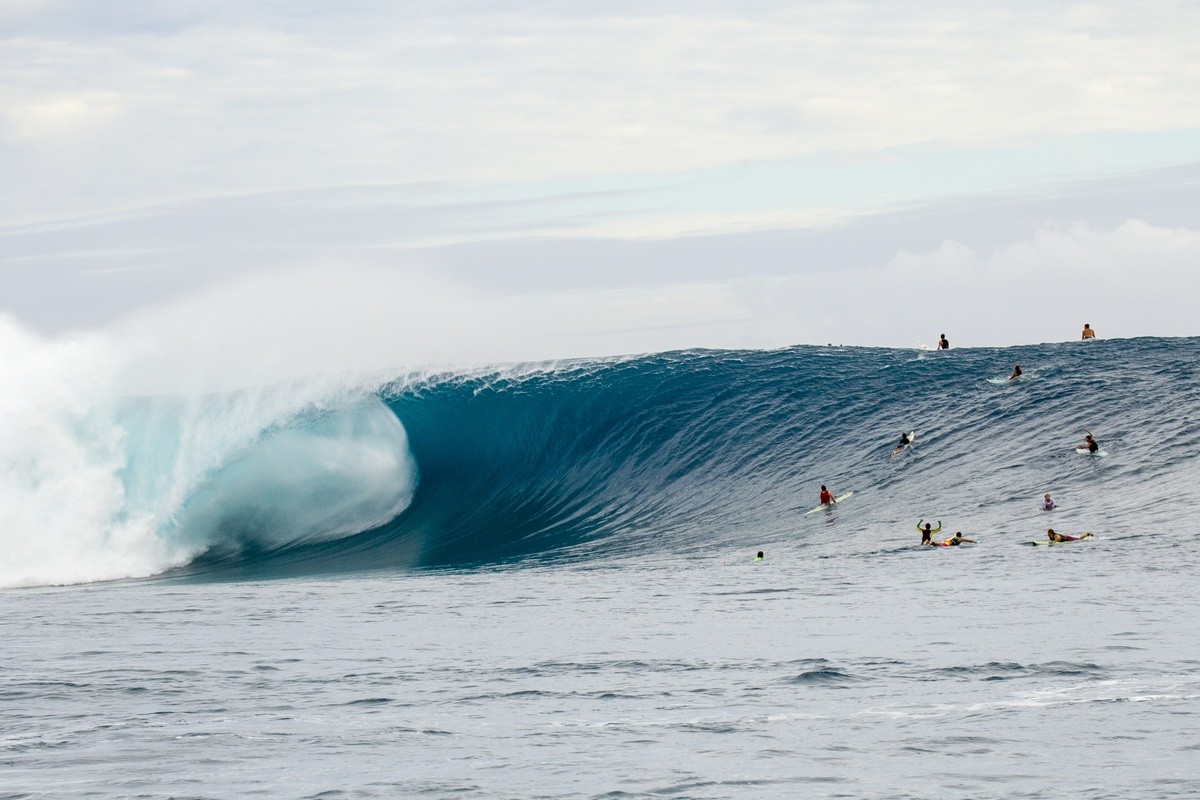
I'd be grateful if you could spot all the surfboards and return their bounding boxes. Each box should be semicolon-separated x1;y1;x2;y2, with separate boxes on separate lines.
1076;448;1109;457
803;491;853;514
891;431;915;457
986;376;1026;384
920;344;937;351
1035;540;1069;544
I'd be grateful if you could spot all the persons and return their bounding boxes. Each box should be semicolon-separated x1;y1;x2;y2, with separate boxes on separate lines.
1009;366;1022;380
922;532;976;546
1079;430;1098;454
937;334;949;350
1048;529;1094;542
916;520;941;545
1082;324;1095;340
820;485;836;505
892;433;910;457
1042;494;1056;511
754;551;763;561
